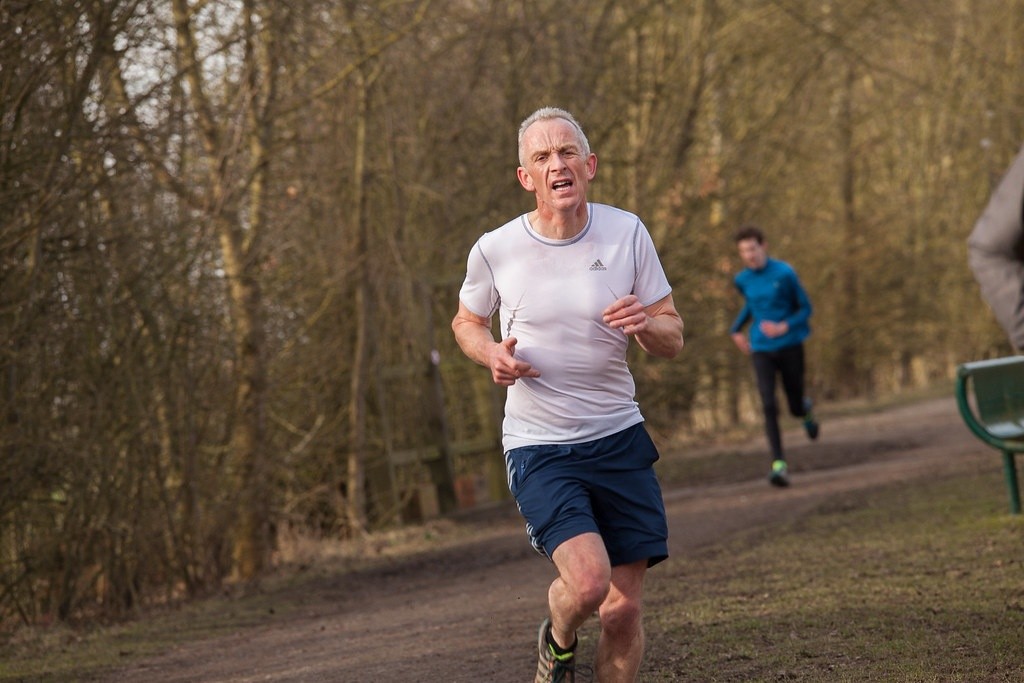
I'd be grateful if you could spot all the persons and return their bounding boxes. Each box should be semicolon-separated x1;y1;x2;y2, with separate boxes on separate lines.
966;147;1024;356
730;228;819;486
453;108;683;683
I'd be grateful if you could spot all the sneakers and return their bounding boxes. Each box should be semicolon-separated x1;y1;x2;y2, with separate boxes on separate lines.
534;616;581;683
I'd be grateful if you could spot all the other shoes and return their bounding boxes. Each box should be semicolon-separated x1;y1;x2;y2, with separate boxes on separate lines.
767;460;792;487
802;400;819;440
